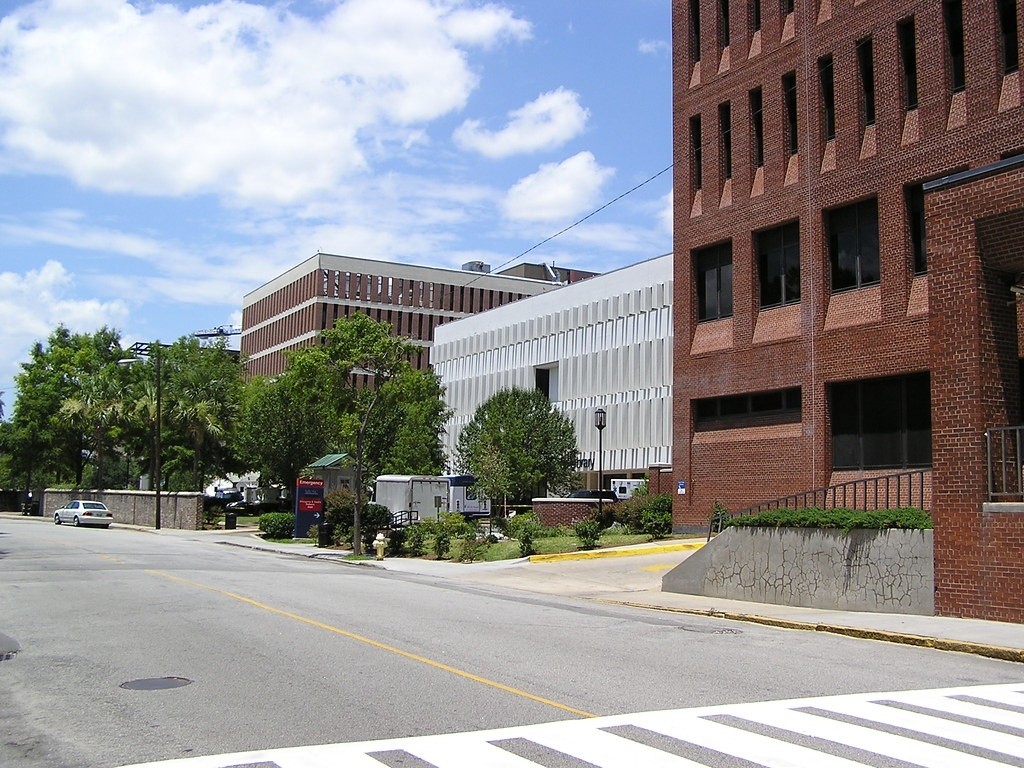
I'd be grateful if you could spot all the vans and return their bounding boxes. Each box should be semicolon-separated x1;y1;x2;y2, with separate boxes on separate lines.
216;488;245;504
567;490;619;503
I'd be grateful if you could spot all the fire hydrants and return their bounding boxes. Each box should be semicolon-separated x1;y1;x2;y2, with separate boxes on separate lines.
374;533;387;560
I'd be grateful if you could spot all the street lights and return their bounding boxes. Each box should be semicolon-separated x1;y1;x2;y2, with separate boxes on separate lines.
594;408;607;516
118;359;163;529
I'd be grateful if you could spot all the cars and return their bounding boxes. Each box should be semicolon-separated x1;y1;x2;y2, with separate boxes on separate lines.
226;500;283;515
53;501;113;530
